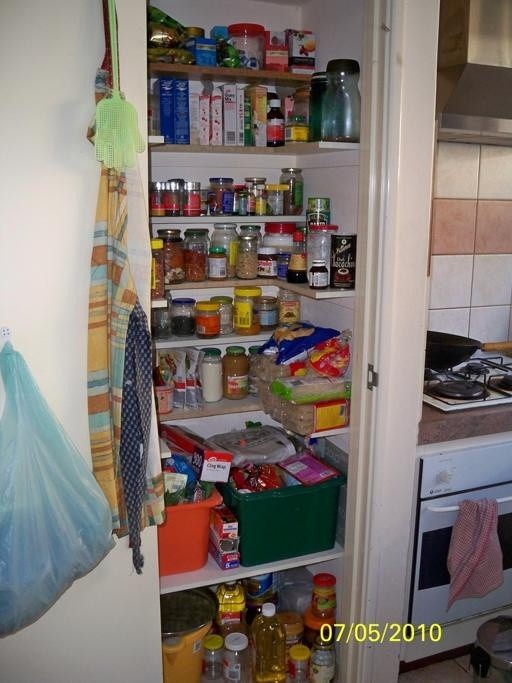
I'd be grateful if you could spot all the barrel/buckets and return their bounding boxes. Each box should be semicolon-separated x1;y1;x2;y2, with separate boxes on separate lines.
215;580;249;637
159;588;218;683
249;602;288;683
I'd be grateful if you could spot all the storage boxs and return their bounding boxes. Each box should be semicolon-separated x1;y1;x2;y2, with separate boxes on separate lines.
213;432;346;567
157;487;224;577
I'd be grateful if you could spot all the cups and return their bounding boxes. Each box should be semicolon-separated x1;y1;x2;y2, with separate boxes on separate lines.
336;267;350;281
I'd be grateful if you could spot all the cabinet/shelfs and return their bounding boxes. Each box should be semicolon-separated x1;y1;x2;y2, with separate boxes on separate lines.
119;1;395;683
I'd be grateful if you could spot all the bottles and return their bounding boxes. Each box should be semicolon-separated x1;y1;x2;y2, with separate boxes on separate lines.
226;23;267;70
292;87;310;124
210;223;338;290
310;70;347;139
322;57;362;141
237;191;247;215
266;99;285;147
169;296;197;337
254;183;267;215
209;295;233;334
248;344;266;396
202;570;337;682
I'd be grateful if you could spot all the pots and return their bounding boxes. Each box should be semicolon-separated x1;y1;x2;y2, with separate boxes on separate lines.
425;328;482;371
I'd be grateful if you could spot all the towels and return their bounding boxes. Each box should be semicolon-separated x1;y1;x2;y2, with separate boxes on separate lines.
446;498;504;611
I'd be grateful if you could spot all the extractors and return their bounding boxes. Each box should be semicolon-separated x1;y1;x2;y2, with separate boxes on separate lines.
434;0;512;145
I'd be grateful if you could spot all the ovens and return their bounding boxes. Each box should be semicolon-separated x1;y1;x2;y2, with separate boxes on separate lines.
405;430;512;668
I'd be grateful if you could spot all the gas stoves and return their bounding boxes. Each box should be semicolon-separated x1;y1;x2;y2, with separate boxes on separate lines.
425;354;511;413
